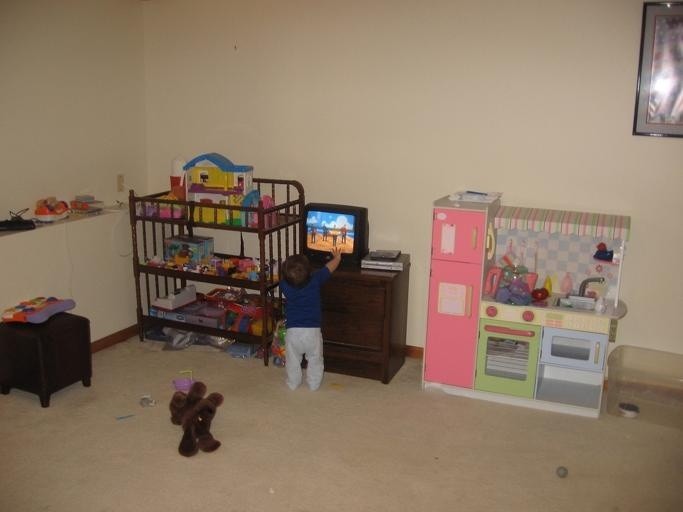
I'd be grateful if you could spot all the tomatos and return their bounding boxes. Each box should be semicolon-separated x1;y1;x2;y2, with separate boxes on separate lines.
532;288;549;300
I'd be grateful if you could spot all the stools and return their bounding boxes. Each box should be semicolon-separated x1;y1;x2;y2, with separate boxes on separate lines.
0;311;92;407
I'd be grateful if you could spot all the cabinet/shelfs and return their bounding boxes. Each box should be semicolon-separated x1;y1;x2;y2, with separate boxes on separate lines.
301;253;411;384
128;174;305;367
421;192;500;390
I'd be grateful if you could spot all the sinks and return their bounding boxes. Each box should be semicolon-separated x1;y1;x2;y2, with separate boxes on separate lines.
553;293;611;316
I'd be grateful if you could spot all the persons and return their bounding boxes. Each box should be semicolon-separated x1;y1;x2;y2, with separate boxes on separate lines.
331;224;337;246
340;224;346;244
279;246;341;392
321;222;327;241
308;223;316;243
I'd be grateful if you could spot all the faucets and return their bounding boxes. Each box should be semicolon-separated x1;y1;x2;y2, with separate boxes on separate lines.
578;276;604;296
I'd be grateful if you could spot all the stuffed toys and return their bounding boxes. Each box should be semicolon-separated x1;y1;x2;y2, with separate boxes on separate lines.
168;380;223;456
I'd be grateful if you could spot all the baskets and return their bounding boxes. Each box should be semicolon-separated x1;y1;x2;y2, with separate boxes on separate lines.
207;289;235;306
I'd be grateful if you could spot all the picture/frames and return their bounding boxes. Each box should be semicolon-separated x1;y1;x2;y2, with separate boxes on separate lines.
632;1;683;138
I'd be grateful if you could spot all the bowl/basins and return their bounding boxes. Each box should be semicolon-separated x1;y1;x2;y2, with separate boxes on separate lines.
173;377;196;391
503;264;528;287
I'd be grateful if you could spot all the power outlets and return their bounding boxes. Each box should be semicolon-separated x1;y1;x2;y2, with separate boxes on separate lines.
117;175;125;192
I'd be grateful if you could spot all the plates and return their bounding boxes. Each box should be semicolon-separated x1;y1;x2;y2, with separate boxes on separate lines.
34;210;71;222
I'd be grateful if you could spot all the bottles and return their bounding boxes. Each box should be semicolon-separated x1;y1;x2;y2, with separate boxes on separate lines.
595;296;606;315
544;275;552;297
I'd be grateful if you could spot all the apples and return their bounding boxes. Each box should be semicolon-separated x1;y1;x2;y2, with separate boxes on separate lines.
37;197;57;210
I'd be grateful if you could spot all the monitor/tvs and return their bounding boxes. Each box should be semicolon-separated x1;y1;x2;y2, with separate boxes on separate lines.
302;203;369;268
